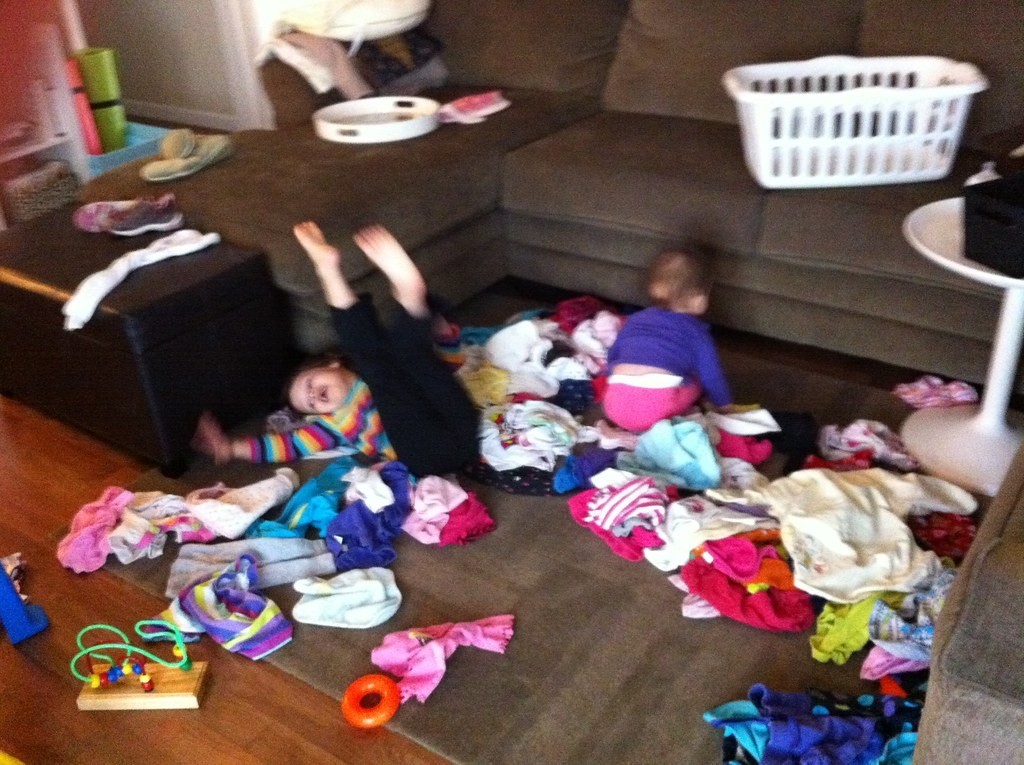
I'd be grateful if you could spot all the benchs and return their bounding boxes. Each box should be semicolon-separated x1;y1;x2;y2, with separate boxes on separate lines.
0;197;288;478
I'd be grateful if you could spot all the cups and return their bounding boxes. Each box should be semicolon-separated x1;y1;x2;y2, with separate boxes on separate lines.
65;47;129;156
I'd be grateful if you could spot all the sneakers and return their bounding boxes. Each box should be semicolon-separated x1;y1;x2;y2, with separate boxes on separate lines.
73;200;138;233
109;192;185;237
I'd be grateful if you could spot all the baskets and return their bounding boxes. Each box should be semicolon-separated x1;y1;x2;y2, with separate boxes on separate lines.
722;53;986;192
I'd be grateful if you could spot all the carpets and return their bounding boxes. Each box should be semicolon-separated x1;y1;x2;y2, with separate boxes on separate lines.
52;293;990;765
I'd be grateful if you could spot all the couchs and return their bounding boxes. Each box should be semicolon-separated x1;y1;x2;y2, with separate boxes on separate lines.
72;0;1024;391
912;444;1024;765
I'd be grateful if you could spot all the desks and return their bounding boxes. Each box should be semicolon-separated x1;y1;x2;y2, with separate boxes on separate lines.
901;195;1024;498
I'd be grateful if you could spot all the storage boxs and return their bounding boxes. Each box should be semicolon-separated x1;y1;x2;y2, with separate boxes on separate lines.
85;122;173;179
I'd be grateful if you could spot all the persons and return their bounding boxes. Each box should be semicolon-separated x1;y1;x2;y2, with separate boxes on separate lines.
603;248;730;431
191;223;480;478
